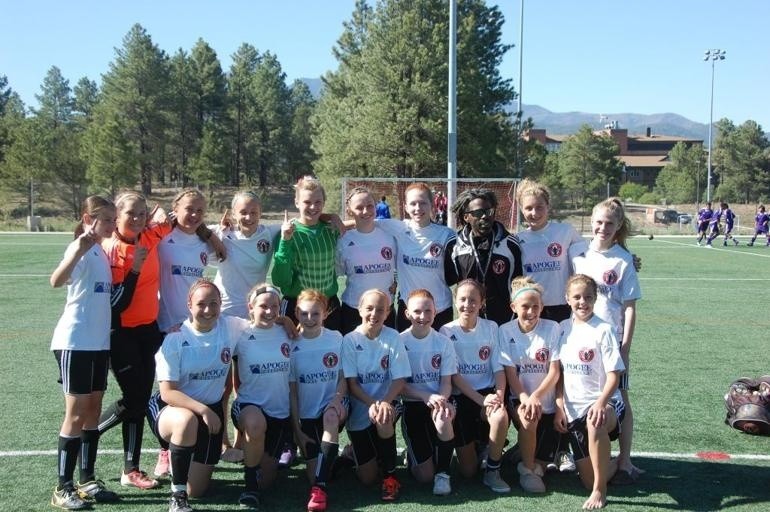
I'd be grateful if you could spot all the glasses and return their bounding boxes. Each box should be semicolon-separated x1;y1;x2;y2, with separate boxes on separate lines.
464;206;495;218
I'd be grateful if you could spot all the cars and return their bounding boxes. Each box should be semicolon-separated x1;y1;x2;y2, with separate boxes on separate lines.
678;213;692;224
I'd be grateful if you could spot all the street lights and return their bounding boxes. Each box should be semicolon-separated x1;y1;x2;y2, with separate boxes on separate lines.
703;48;726;203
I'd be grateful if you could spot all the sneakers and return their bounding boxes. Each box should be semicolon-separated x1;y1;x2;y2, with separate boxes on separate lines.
610;467;639;484
51;481;89;510
381;476;401;500
238;489;260;510
220;446;244;463
697;237;770;249
279;443;297;465
401;444;576;495
345;443;354;460
154;448;169;476
169;490;193;512
76;477;118;503
307;485;328;512
121;468;159;489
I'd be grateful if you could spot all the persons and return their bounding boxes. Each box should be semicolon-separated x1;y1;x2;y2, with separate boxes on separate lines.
146;274;629;511
146;175;645;476
696;203;770;248
48;189;228;510
